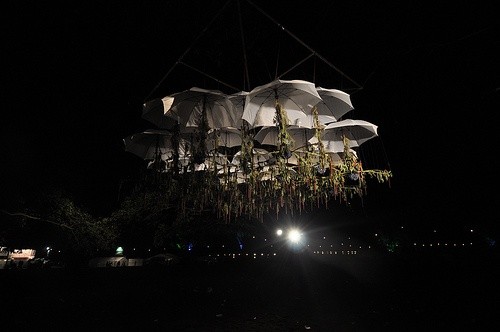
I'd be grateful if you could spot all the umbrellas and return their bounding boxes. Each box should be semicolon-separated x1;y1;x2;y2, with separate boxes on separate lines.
122;78;381;192
